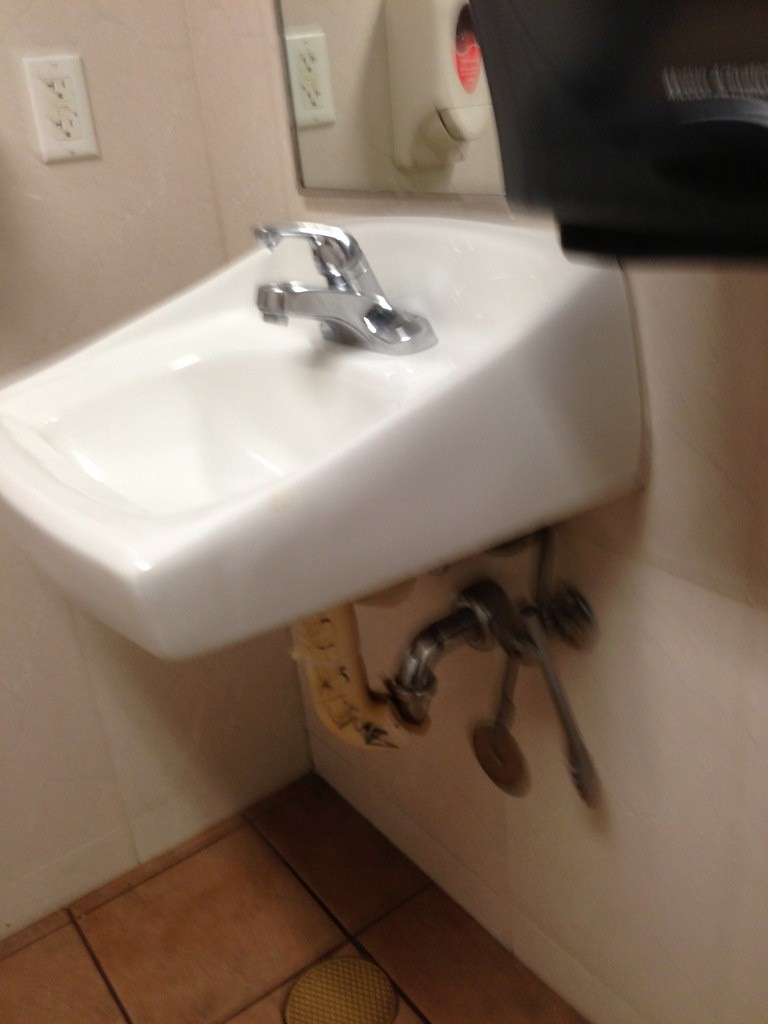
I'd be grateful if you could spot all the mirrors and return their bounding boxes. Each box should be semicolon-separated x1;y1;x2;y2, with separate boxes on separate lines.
276;0;506;199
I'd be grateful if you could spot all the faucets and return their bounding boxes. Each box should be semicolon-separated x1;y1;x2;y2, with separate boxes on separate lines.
252;222;438;356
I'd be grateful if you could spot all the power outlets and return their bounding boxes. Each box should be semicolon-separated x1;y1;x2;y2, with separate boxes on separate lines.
285;31;335;127
24;53;98;163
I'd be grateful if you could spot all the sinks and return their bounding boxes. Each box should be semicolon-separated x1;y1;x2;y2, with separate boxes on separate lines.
2;211;644;660
